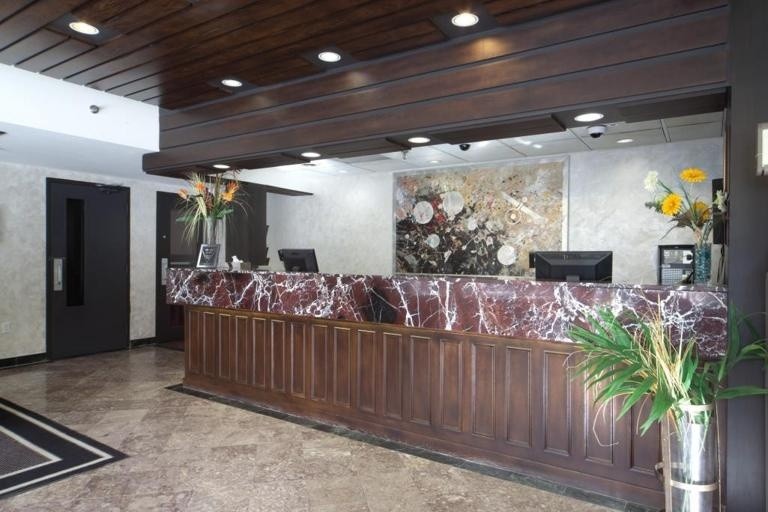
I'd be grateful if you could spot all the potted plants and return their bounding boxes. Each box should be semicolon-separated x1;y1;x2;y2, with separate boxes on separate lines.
557;298;767;512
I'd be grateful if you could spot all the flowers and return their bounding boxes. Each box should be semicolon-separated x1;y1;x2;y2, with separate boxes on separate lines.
172;165;253;249
644;166;731;249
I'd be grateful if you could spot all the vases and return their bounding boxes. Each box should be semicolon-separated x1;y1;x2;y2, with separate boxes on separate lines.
692;244;711;285
204;216;218;245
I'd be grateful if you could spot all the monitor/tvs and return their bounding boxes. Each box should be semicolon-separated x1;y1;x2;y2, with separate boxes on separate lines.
278;249;319;272
528;250;613;283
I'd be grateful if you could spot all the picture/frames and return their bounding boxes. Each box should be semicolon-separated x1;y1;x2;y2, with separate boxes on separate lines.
195;243;221;269
389;152;572;281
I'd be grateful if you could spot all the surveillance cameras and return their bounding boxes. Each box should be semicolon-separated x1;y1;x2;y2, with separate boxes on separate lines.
459;144;471;151
587;127;606;140
